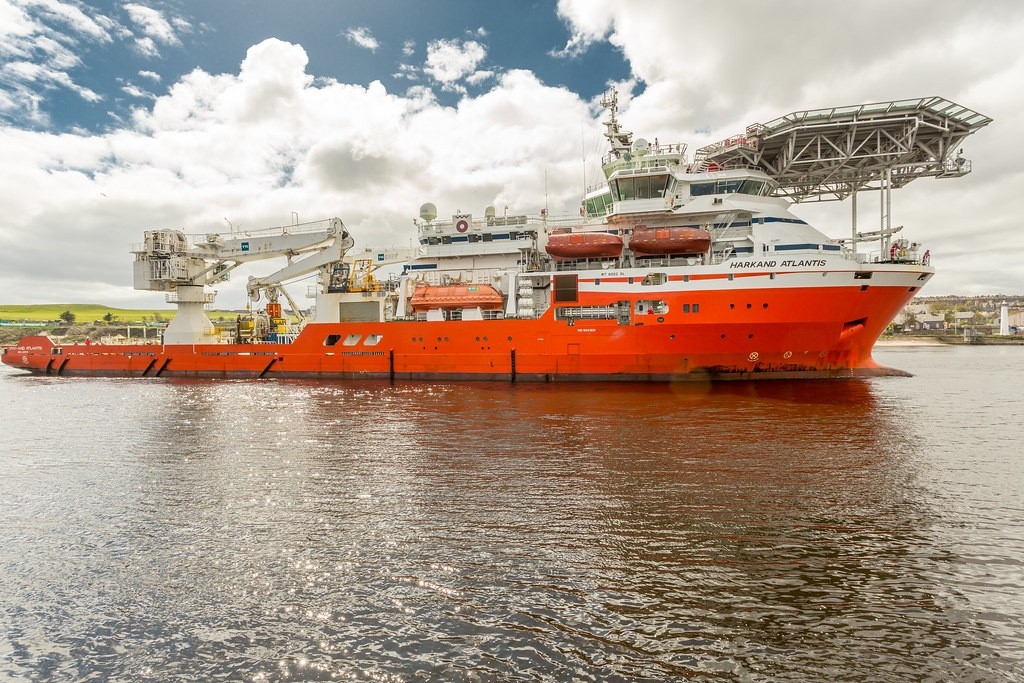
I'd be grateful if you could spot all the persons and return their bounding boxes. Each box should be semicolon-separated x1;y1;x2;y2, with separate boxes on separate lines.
922;250;930;265
356;267;365;287
890;240;902;264
85;336;91;345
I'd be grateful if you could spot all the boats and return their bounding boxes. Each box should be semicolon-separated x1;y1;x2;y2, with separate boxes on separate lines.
2;83;994;382
